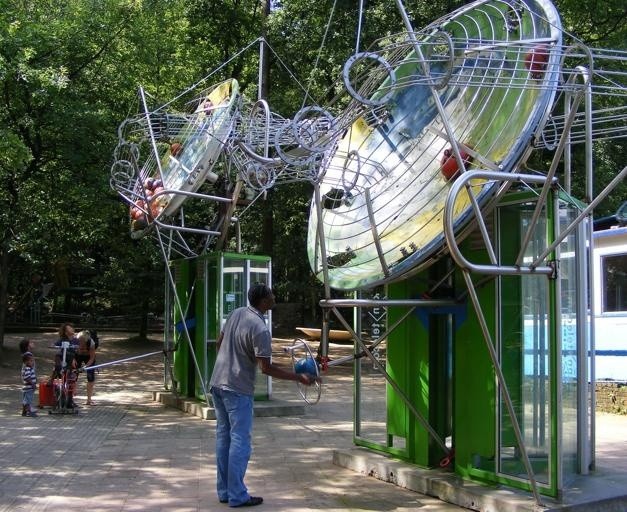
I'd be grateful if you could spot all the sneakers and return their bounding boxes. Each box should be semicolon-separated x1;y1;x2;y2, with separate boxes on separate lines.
22;410;38;417
219;495;264;508
67;401;98;408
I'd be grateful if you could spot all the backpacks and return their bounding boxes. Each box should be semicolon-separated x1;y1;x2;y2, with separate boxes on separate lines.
86;328;99;351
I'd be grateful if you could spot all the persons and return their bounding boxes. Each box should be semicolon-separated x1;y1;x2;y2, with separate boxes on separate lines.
207;282;316;508
19;323;96;418
